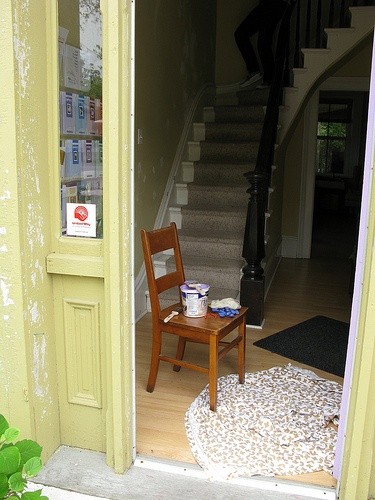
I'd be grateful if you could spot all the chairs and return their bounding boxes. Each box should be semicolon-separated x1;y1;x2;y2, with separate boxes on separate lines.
139;221;248;412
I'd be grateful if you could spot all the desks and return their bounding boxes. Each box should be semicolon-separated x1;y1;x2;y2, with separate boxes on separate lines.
314;178;348;222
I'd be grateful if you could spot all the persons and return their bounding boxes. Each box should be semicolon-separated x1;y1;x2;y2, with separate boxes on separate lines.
234;0;282;90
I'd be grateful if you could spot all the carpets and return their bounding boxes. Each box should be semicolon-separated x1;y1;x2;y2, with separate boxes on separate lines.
253;315;350;380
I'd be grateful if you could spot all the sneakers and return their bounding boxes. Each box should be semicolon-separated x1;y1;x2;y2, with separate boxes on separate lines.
255;78;270;89
239;71;262;88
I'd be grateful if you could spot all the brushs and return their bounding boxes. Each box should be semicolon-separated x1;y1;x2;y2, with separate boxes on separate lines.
163;306;184;323
184;279;206;296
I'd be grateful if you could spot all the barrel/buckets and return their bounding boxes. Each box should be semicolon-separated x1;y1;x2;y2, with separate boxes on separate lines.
180;284;210;318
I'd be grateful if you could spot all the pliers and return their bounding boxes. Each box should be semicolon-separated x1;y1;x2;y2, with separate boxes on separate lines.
204;309;218;320
